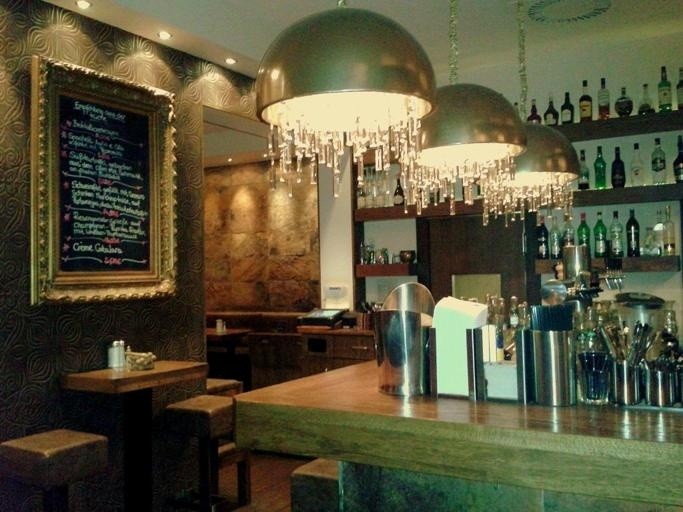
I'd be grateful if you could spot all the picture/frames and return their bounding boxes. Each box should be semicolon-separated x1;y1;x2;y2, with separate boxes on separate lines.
28;48;180;319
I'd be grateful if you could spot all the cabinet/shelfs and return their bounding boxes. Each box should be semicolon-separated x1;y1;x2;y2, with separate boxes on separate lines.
349;143;428;276
525;109;682;302
297;322;376;370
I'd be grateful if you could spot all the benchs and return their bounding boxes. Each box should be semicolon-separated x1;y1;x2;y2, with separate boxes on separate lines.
205;309;303;356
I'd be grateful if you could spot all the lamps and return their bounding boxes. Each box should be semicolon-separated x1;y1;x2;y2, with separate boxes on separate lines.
254;1;437;198
405;0;527;220
488;0;580;233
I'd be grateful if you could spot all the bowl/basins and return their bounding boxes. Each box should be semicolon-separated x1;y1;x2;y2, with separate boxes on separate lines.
398;249;417;263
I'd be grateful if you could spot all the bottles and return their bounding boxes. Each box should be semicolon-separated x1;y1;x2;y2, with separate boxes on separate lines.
577;133;683;190
514;63;683;124
358;166;389;208
461;293;530;359
112;340;131;366
536;204;676;259
392;178;404;207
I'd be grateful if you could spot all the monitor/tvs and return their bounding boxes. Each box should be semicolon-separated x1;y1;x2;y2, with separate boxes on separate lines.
297;308;350;325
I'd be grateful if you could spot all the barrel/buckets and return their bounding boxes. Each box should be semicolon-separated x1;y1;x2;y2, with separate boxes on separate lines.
371;280;437;401
613;292;665;346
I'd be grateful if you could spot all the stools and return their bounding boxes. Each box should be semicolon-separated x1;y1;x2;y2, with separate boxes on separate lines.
202;375;244;404
167;392;250;512
1;426;111;512
285;458;342;511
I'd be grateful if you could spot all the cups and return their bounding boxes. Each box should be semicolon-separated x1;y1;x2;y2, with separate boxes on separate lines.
215;319;223;333
571;288;683;409
